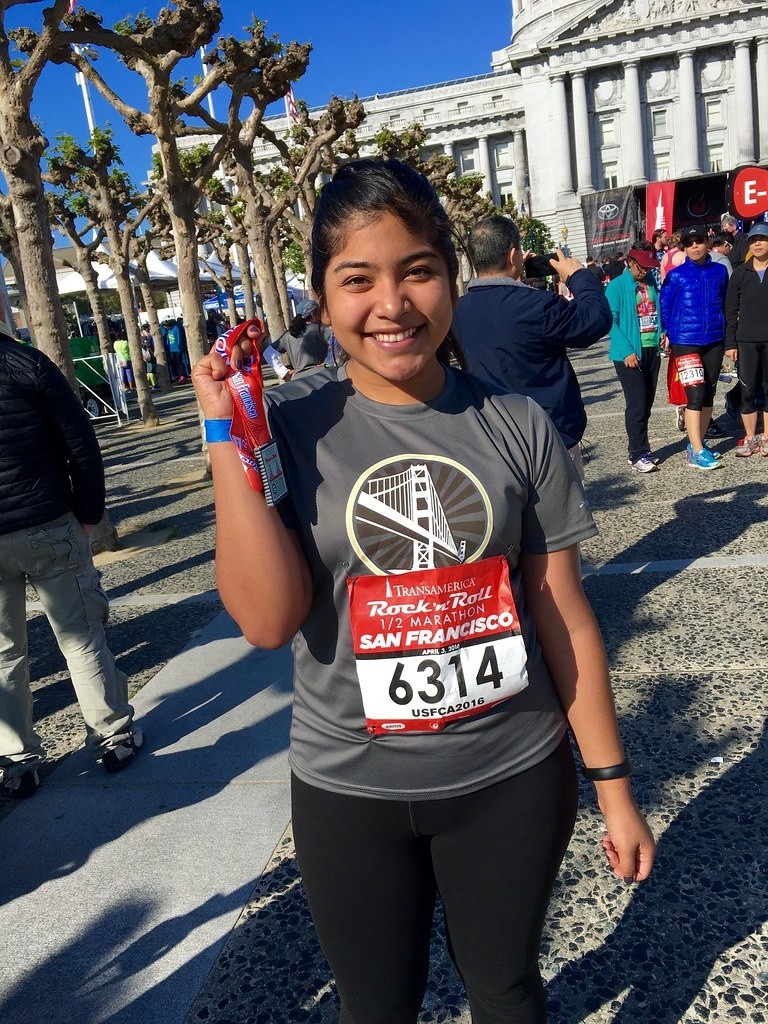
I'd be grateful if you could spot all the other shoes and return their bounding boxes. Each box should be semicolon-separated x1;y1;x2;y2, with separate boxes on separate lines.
180;375;187;382
152;386;161;391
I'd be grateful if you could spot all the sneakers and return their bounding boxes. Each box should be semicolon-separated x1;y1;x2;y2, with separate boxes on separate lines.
0;768;40;799
102;728;143;774
760;435;768;456
686;443;721;459
628;452;659;472
725;392;737;420
736;436;760;456
705;423;727;436
676;406;687;432
686;448;723;470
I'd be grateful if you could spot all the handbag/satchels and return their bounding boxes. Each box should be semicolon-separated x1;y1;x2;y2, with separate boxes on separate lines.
142;348;151;363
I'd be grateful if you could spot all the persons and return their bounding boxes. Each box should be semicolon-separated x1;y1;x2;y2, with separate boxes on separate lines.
262;300;340;382
0;288;147;800
190;156;655;1024
11;330;26;345
114;309;241;393
449;214;614;482
567;215;768;473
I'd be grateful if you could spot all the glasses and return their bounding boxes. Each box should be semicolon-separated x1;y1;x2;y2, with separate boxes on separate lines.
725;241;733;250
683;237;708;247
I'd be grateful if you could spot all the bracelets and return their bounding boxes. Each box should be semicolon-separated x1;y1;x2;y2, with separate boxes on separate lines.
581;757;633;781
201;419;233;443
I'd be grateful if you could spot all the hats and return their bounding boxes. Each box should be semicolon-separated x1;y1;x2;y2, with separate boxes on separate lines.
681;225;708;245
745;224;768;240
297;299;319;318
629;249;660;267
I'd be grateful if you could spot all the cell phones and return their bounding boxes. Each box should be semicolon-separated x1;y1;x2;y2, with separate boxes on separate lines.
525;253;560;279
